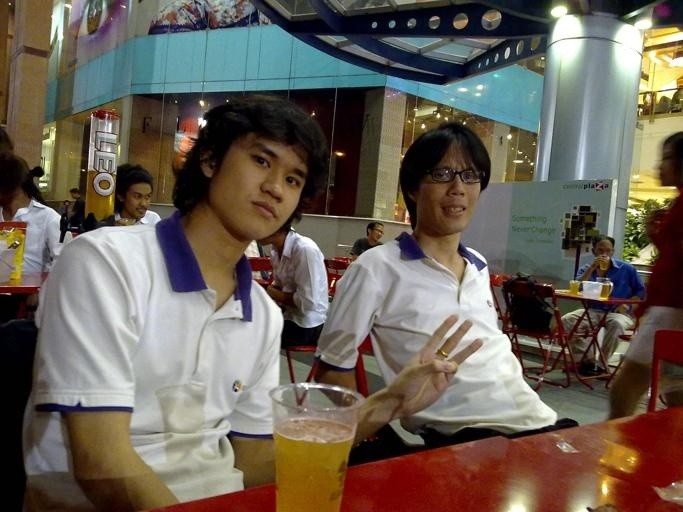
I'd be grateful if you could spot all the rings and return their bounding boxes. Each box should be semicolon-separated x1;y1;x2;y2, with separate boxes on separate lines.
438;350;448;358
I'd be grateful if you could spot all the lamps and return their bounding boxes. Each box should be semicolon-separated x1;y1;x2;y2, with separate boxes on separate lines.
634;7;654;32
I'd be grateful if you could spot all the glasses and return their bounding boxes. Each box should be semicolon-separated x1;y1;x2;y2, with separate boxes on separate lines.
431;166;487;184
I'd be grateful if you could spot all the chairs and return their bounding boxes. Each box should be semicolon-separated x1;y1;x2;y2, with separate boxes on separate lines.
502;279;571;392
638;96;681;116
590;284;649;388
324;258;356;296
488;273;552;375
647;329;683;411
0;319;43;512
281;273;343;408
352;332;378;444
246;256;276;293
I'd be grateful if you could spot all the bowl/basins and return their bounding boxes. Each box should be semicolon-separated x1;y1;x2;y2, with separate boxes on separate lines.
582;280;604;298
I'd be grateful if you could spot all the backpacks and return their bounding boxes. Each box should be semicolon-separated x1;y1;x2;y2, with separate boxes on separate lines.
503;271;559;330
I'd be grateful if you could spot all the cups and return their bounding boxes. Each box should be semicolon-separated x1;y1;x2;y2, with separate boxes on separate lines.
568;279;581;296
268;381;365;512
596;276;613;300
600;254;610;271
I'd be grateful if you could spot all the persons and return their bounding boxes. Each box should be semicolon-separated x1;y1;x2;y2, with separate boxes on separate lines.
257;209;328;348
1;150;73;286
605;129;682;424
312;119;577;455
17;93;484;512
69;187;85;223
348;222;383;260
559;235;645;376
